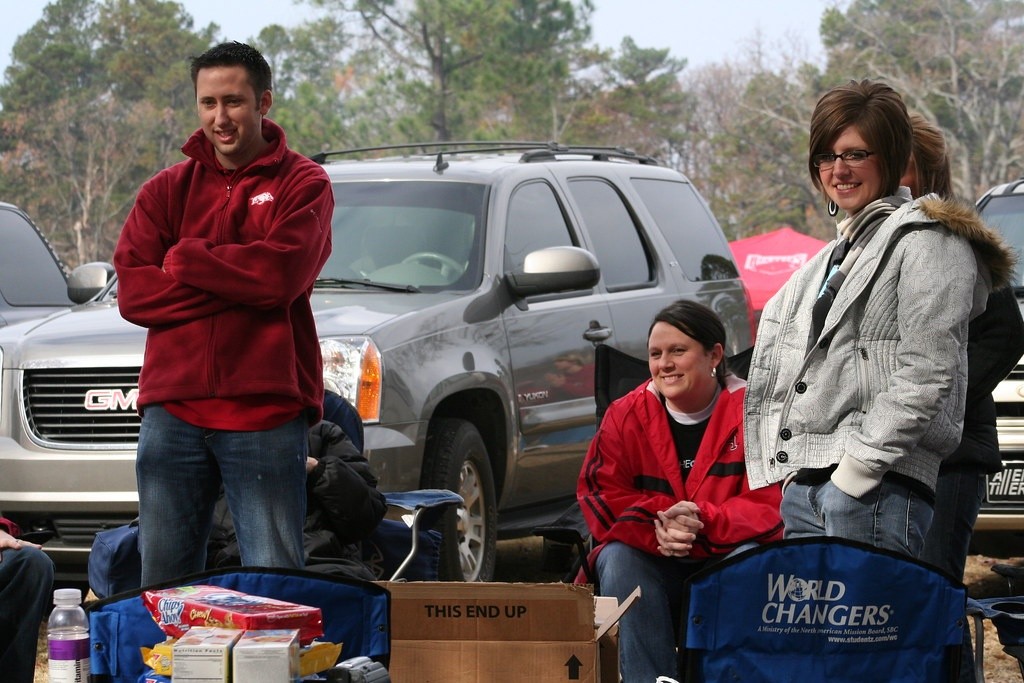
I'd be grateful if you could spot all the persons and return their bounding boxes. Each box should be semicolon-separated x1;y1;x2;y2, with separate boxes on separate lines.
744;82;1024;683
0;515;54;683
113;41;334;589
544;354;594;402
204;417;380;586
576;300;784;683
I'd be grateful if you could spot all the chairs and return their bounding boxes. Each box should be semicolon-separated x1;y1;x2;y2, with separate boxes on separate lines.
533;343;753;594
654;535;1024;683
82;389;465;605
83;566;391;683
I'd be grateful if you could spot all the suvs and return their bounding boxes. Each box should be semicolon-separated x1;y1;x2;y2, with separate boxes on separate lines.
0;141;760;583
970;178;1024;535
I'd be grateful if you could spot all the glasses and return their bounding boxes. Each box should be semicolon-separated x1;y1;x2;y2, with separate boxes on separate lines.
810;148;876;168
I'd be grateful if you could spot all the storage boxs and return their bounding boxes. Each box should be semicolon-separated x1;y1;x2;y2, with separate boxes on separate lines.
171;626;245;683
232;628;301;683
371;579;643;683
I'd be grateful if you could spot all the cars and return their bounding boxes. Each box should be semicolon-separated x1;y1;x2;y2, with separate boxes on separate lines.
0;200;116;333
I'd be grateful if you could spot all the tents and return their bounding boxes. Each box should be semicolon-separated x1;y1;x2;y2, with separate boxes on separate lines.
728;227;832;314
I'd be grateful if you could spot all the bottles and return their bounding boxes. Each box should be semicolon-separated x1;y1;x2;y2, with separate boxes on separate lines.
47;589;90;683
